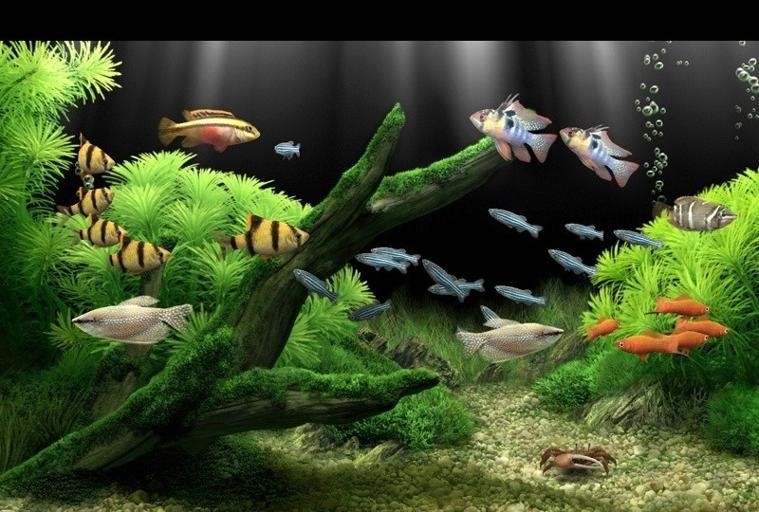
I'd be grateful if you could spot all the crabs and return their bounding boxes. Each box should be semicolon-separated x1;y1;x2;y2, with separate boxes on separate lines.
538;443;618;477
